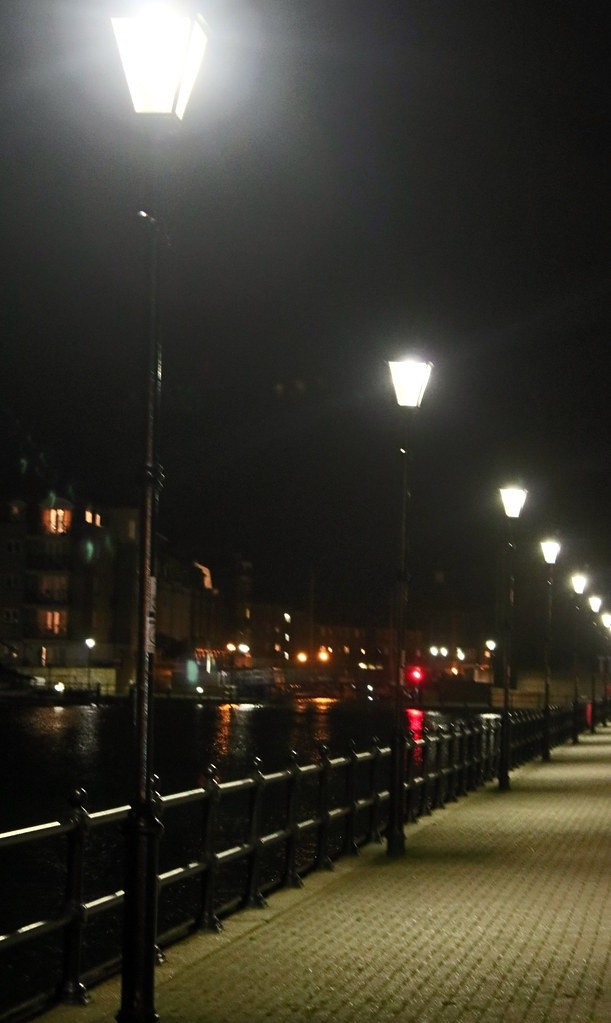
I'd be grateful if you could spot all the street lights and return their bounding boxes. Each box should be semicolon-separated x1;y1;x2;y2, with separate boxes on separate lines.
384;338;430;854
101;1;182;1015
537;538;552;765
567;573;610;738
497;474;524;795
